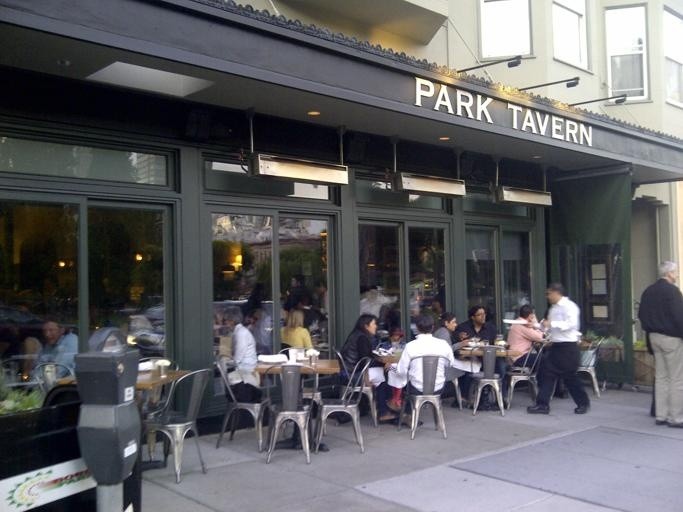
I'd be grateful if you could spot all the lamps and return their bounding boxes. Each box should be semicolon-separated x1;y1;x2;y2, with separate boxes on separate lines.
230;255;243;272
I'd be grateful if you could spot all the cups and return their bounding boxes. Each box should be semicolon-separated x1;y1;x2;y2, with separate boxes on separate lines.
482;340;489;346
310;355;319;365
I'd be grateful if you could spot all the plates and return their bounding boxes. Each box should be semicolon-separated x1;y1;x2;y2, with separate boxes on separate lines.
138;360;170;370
504;320;528;324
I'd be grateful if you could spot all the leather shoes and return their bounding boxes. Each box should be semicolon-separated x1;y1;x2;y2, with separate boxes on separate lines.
528;403;550;414
574;403;590;414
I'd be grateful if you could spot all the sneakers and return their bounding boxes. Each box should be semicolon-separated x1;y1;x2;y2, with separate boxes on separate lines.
379;412;399;423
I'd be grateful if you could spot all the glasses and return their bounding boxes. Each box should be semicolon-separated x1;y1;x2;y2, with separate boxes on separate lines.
473;313;487;317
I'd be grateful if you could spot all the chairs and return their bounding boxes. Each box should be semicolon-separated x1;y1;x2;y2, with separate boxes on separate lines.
467;345;508;416
138;357;178;467
335;351;378;427
575;337;607;399
398;356;451;439
311;356;371;453
35;363;76;391
507;341;545;409
279;347;327;437
215;354;271;453
262;364;319;464
140;367;213;484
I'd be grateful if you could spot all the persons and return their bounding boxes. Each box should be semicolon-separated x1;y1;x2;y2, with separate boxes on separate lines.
283;273;307;311
451;305;507;409
433;312;482;409
220;306;257;371
32;318;78;379
637;261;683;428
336;314;400;424
527;282;590;415
280;310;313;351
246;280;266;313
396;314;454;429
302;279;328;328
360;285;398;319
507;304;543;388
